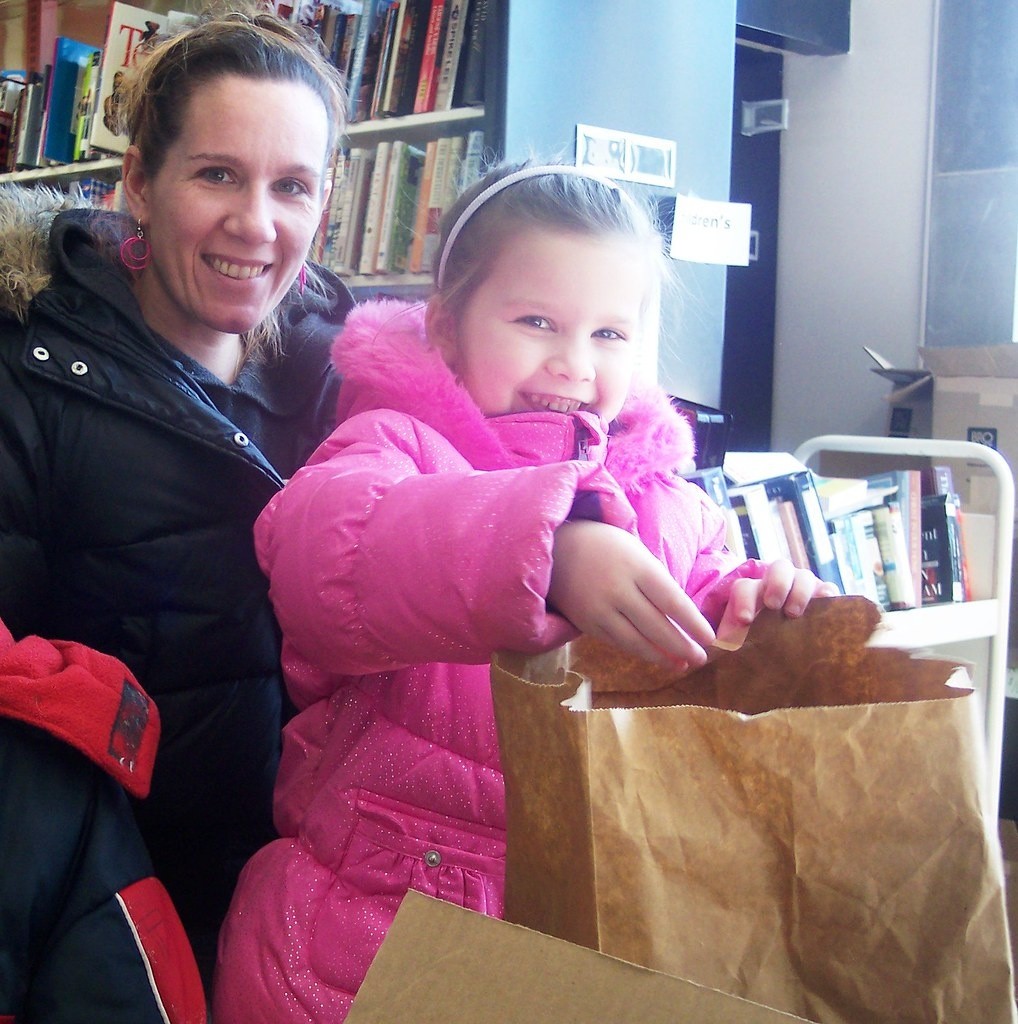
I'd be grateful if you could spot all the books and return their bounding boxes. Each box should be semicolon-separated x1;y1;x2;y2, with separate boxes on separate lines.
0;0;486;276
682;466;975;611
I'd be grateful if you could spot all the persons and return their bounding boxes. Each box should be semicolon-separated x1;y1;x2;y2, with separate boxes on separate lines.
209;162;841;1024
0;0;355;1024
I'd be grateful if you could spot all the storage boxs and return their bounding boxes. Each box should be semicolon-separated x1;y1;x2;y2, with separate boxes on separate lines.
861;341;1018;525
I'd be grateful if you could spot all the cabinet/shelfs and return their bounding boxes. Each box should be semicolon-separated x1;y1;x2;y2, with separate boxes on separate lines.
0;100;485;304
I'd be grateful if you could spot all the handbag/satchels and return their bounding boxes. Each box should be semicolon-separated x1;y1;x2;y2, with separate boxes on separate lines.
488;596;1017;1024
